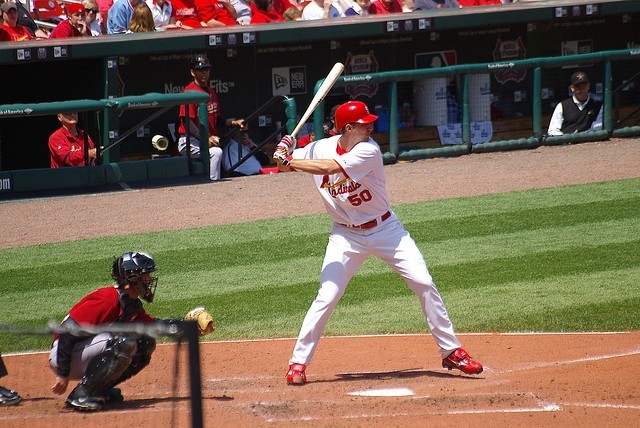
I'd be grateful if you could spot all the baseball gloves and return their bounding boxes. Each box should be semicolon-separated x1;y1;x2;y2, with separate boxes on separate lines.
184;307;216;336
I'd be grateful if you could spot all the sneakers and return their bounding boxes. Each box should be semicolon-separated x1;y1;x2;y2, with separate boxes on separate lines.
440;349;482;374
101;386;125;403
64;387;104;411
285;363;306;385
0;385;23;404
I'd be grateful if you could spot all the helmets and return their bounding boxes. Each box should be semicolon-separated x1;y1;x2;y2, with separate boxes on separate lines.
335;101;378;133
110;251;158;303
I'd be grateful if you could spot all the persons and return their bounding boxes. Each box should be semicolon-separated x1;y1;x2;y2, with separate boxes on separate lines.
177;54;247;181
108;0;142;36
5;0;48;39
146;1;182;32
126;2;156;34
325;103;344;137
48;4;86;40
169;1;208;30
82;0;103;37
0;2;47;41
268;101;483;386
297;130;316;148
0;350;23;409
548;72;605;135
222;122;270;175
194;0;237;28
48;112;104;168
48;251;214;411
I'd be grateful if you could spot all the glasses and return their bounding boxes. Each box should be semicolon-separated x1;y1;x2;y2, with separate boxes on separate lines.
85;8;99;14
239;130;248;134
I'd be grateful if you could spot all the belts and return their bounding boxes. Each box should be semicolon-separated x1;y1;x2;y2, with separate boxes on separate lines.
333;211;391;228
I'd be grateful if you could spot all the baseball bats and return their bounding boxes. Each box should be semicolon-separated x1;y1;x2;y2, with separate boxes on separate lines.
272;62;345;164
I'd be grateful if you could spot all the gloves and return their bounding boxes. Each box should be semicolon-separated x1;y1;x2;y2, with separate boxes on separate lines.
273;146;292;167
278;135;296;155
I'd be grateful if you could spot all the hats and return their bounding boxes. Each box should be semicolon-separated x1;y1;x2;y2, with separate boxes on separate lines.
1;1;17;13
68;3;85;15
190;55;213;70
570;72;588;85
